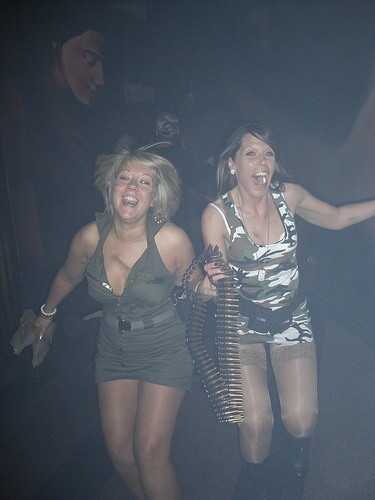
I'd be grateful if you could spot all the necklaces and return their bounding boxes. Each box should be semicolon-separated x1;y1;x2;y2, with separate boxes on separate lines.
237;190;269;283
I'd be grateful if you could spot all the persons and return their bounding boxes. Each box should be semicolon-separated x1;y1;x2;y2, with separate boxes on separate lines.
202;123;375;500
35;141;216;500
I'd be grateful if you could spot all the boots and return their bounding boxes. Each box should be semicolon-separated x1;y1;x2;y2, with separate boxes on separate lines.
270;419;311;500
233;453;271;500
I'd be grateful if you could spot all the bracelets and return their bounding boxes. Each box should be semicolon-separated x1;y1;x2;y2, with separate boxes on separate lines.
39;304;57;320
191;279;213;303
